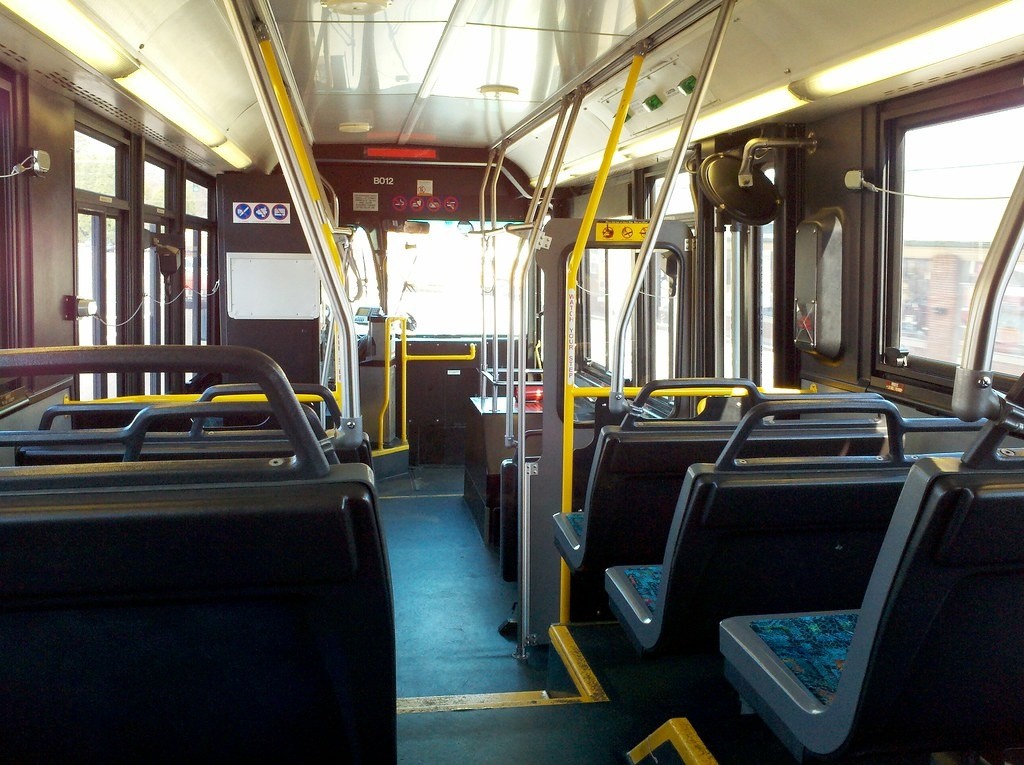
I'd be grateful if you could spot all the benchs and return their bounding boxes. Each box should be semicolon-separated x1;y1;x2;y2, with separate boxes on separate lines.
0;344;397;765
497;378;1024;764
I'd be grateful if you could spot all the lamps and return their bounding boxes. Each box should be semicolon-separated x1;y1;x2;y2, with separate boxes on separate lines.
0;0;253;170
76;299;97;317
528;0;1024;192
